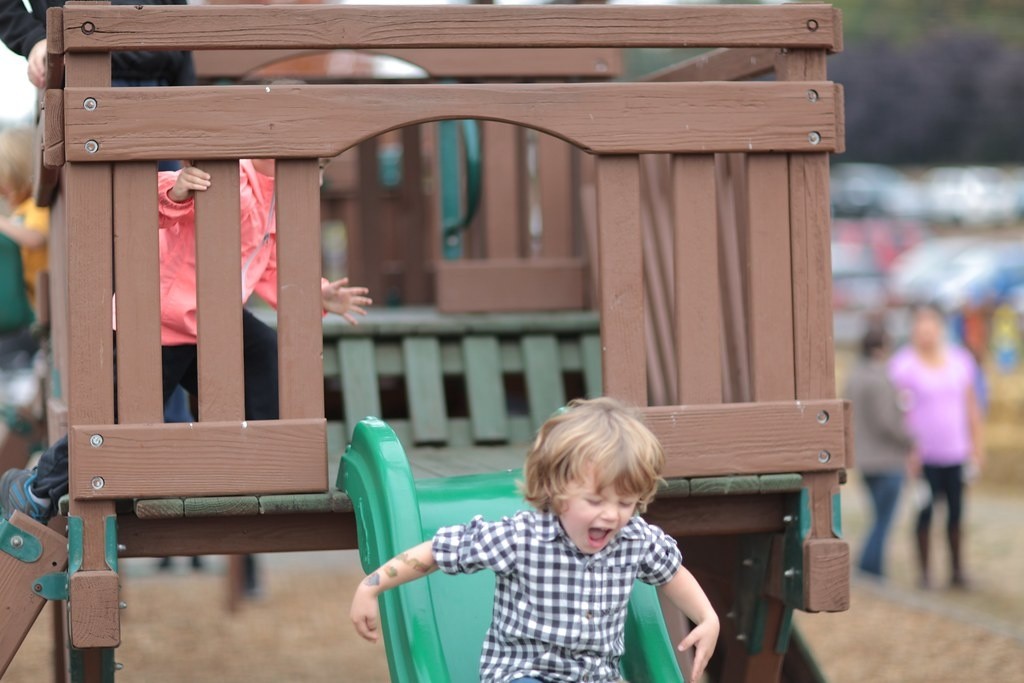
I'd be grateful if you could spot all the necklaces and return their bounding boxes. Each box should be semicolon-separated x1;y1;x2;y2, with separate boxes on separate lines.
349;397;721;683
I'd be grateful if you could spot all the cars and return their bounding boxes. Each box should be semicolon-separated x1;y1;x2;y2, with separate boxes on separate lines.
820;152;1022;356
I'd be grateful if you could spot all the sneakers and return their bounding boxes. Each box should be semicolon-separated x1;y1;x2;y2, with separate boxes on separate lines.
2;469;53;529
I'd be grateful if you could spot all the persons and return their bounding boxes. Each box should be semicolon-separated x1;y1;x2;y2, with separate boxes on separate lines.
884;300;989;589
0;129;54;368
1;158;375;527
846;326;916;581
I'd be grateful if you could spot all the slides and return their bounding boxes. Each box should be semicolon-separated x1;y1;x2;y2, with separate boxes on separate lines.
335;416;688;683
